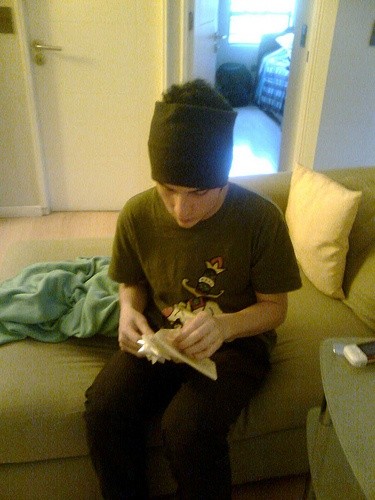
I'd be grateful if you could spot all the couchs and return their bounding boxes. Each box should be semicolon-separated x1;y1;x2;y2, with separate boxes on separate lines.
0;166;374;500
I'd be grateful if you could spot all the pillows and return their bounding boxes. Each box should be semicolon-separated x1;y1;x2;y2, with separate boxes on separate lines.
285;162;363;301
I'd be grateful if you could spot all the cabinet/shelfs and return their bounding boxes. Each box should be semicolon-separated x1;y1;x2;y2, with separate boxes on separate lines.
303;337;373;499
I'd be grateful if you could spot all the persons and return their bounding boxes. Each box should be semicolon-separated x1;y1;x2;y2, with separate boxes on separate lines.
82;76;304;499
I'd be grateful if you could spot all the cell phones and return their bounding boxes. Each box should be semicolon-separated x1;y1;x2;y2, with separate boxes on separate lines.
344;341;375;368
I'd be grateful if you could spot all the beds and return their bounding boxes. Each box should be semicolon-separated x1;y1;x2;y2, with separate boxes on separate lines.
254;42;291;124
